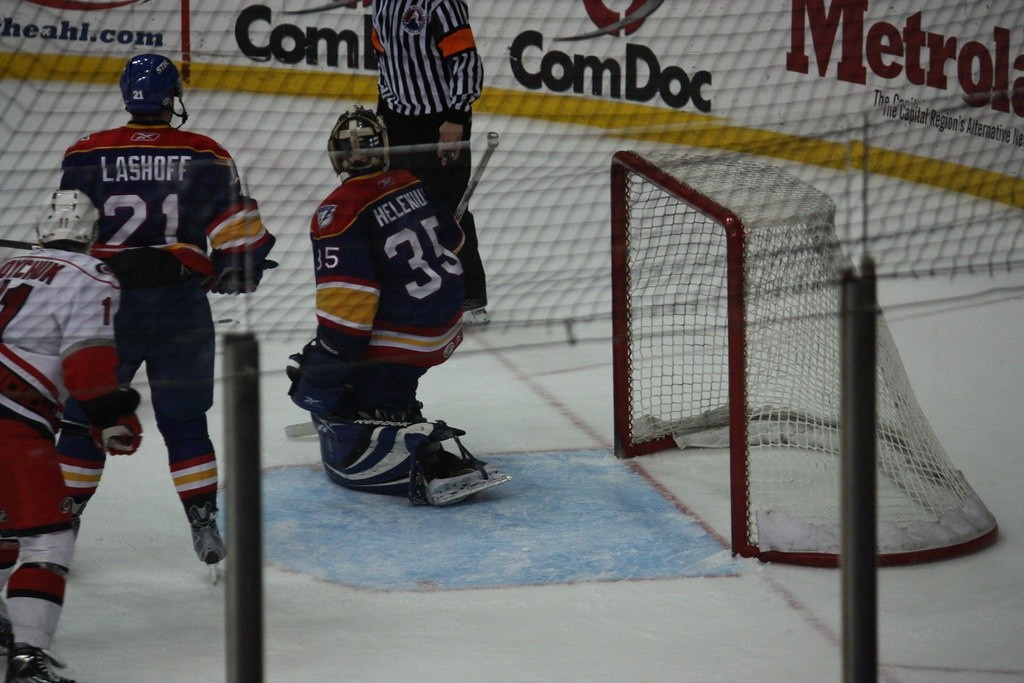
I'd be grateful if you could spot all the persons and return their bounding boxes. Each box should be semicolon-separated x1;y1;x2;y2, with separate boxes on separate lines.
59;54;279;565
373;1;488;324
0;190;143;683
286;107;489;506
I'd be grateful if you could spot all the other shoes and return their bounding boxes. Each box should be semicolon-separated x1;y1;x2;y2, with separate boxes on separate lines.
460;308;489;324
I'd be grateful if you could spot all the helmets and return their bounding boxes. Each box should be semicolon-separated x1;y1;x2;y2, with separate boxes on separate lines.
327;106;391;175
119;54;182;113
36;188;99;244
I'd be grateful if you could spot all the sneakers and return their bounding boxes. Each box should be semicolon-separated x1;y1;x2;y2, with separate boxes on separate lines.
0;615;13;652
6;642;75;683
190;500;226;586
412;448;513;507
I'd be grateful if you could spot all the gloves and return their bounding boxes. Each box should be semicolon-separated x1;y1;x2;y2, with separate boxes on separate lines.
92;407;143;455
209;234;277;294
285;337;349;389
